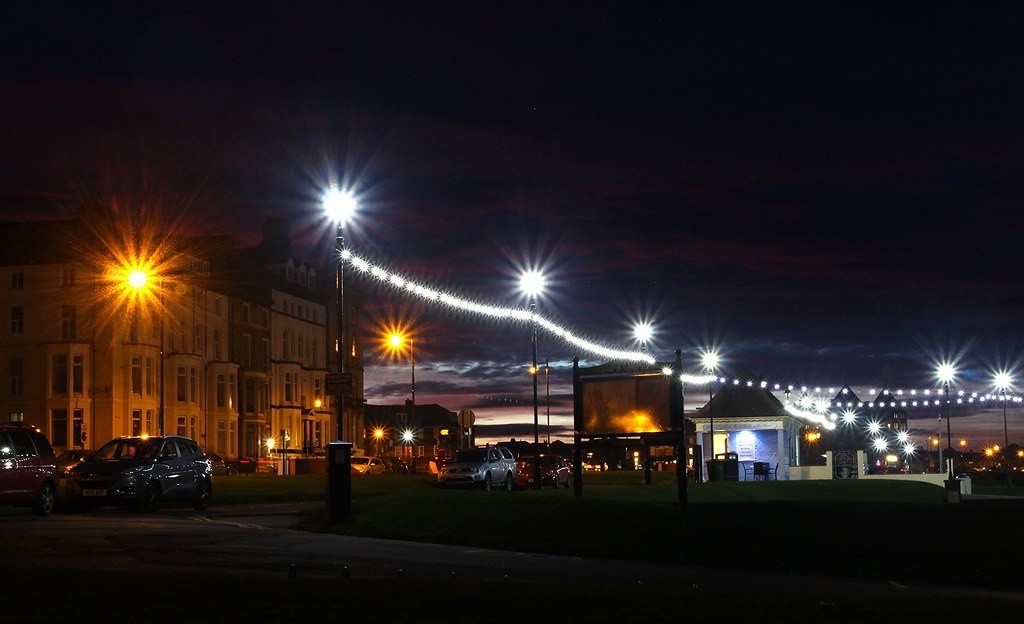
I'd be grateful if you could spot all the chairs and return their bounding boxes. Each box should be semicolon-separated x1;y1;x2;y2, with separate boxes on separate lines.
742;462;779;481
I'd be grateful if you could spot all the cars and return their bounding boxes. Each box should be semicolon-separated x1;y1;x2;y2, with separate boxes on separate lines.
65;435;213;513
0;424;59;516
351;455;408;475
55;449;107;481
521;454;574;490
438;444;518;491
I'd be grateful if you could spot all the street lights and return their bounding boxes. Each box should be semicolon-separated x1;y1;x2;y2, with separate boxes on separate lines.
388;332;415;405
800;394;916;474
783;387;793;406
404;431;413;471
635;322;655;372
375;430;390;454
941;365;954;479
998;374;1012;486
522;269;546;490
321;182;356;512
130;267;165;434
985;444;1000;457
705;351;718;483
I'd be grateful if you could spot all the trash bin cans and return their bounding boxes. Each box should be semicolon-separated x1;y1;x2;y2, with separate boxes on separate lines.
705;453;739;481
956;477;972;495
324;440;353;523
